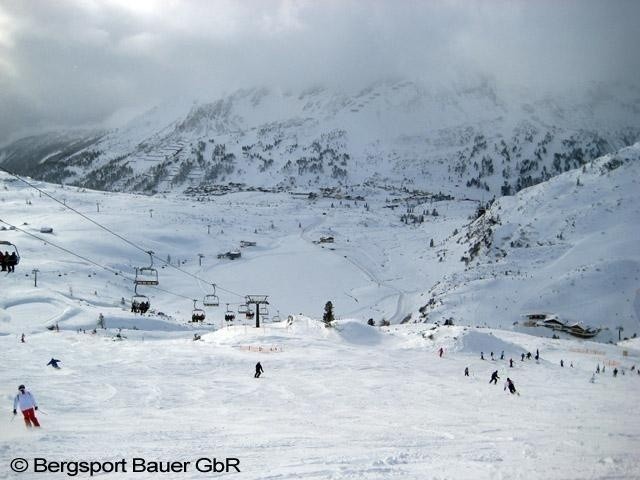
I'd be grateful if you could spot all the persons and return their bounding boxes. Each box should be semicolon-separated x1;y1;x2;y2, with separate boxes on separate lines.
8;251;18;274
130;288;639;400
20;330;26;343
46;357;62;369
0;250;4;273
12;385;40;429
3;249;12;276
55;322;61;332
